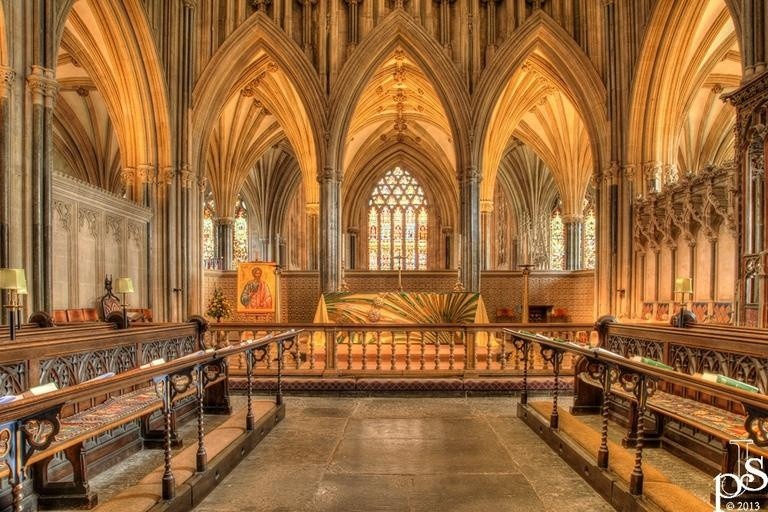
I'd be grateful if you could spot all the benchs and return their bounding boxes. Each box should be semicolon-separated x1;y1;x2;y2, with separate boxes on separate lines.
568;368;767;511
0;371;231;512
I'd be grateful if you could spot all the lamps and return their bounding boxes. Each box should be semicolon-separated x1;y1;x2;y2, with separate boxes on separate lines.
114;277;135;329
672;277;692;328
0;267;28;341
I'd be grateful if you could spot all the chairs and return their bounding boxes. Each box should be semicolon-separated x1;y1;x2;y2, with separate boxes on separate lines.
51;307;153;326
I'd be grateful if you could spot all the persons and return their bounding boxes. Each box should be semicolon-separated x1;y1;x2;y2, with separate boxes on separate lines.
240;267;273;308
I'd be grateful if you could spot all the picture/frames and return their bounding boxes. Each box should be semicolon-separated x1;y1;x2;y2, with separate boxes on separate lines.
236;262;276;314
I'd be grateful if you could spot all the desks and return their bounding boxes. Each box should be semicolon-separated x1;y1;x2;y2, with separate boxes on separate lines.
598;322;766;512
0;322;206;511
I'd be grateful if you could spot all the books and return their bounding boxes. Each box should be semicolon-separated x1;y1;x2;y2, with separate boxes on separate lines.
141;356;165;369
551;336;564;342
18;381;58;397
6;394;24;403
518;328;536;336
633;356;673;371
702;370;759;392
